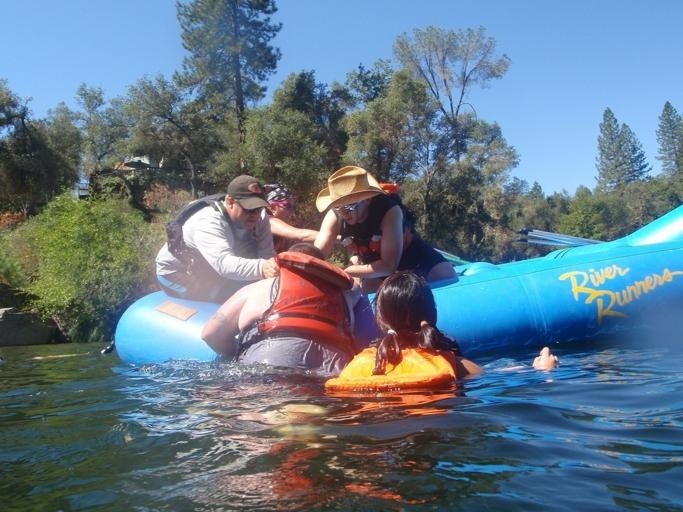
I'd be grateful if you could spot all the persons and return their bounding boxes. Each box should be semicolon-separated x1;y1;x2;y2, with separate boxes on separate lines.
314;165;457;293
260;182;342;250
152;175;278;305
202;242;362;374
325;269;559;394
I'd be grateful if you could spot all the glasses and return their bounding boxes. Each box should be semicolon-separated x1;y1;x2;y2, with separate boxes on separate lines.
335;201;366;211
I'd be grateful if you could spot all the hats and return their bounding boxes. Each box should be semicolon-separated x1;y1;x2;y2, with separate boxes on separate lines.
228;174;268;211
315;164;385;214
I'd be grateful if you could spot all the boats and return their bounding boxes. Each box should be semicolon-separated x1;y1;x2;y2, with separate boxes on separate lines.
112;205;683;368
